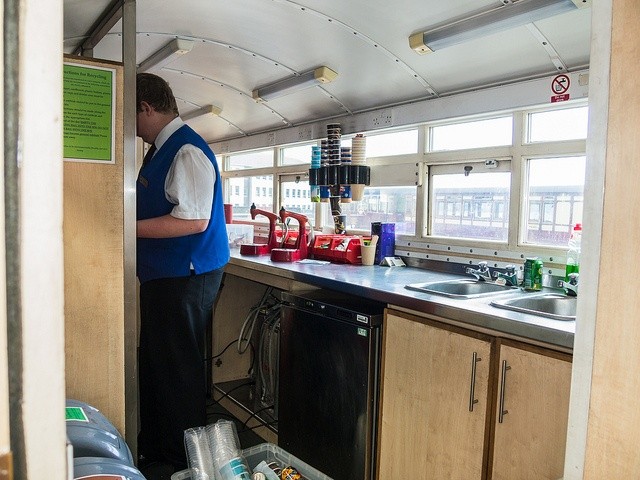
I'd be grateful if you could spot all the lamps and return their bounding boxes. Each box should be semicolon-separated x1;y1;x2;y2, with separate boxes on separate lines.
409;0;578;56
252;65;339;104
181;105;224;123
136;37;194;74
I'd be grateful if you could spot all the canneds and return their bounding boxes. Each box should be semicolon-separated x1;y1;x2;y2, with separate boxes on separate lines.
524;257;543;291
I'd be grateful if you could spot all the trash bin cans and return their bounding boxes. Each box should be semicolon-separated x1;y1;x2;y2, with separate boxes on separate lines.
66;400;134;466
74;457;148;480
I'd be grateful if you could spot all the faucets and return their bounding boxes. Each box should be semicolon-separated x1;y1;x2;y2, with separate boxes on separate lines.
558;271;578;298
493;265;517;286
467;262;492;281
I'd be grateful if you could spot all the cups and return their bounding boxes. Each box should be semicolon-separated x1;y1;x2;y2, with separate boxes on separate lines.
341;147;352;203
311;146;322;202
352;137;365;201
334;215;347;235
182;420;251;480
361;245;376;266
320;140;329;203
224;204;233;224
327;123;342;215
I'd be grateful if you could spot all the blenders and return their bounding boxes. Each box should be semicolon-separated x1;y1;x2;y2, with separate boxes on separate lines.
240;202;290;254
270;205;313;262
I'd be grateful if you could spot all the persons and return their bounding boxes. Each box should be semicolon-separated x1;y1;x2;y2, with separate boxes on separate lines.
136;69;234;479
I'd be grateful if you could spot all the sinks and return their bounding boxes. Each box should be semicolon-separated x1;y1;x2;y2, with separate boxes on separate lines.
504;294;578;320
419;280;517;298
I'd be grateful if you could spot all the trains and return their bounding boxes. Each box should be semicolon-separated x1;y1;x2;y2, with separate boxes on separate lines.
327;187;584;243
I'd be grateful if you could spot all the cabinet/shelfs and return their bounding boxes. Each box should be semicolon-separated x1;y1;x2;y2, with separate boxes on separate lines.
375;307;574;480
